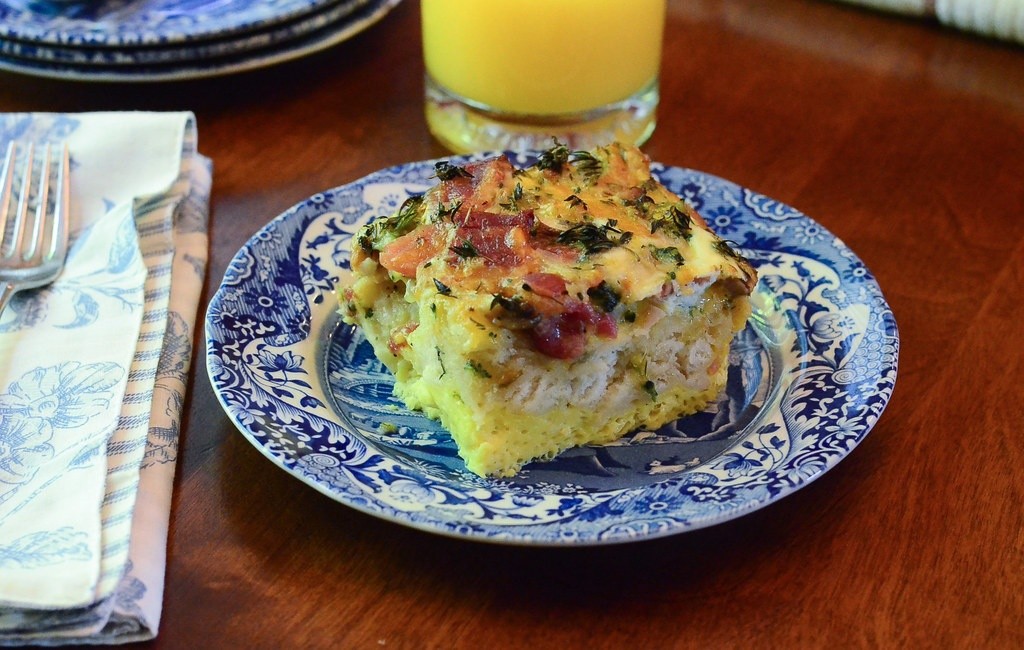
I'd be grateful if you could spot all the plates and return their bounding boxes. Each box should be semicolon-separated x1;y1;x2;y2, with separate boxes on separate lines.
204;154;901;544
0;0;401;83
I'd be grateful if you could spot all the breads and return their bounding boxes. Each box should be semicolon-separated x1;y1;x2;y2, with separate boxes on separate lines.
337;135;757;481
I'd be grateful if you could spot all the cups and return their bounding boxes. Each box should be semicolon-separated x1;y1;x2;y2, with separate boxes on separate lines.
420;0;667;155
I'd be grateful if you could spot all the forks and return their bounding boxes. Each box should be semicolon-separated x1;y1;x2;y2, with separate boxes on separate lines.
0;140;71;318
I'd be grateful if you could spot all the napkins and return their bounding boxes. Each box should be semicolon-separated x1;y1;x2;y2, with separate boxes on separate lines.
0;112;212;647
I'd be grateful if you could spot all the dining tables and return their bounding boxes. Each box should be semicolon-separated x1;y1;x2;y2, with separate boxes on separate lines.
0;0;1024;650
